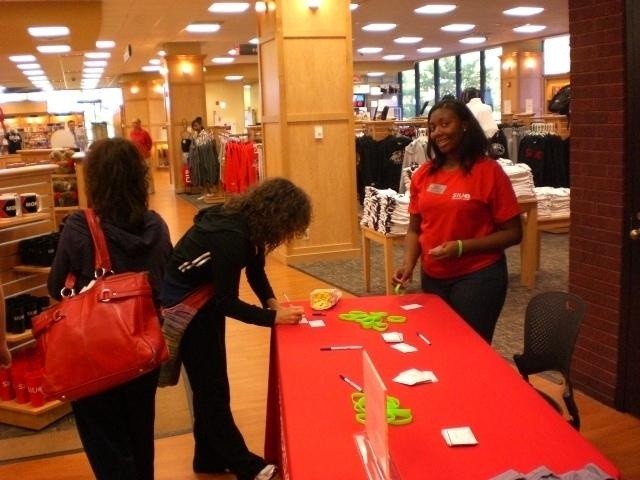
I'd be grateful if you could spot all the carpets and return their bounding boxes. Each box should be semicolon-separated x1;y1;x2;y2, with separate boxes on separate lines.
0;364;195;465
177;192;218;211
288;231;569;385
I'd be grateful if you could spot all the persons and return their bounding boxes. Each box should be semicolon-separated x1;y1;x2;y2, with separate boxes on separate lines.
64;120;83;148
161;174;314;480
7;127;22;153
130;117;156;194
47;136;180;480
190;118;206;138
390;101;523;344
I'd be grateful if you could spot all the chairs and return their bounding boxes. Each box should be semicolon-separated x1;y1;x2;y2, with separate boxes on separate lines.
514;291;586;431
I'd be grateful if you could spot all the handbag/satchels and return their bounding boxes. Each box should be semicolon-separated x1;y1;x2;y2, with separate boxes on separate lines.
155;301;197;390
29;270;172;405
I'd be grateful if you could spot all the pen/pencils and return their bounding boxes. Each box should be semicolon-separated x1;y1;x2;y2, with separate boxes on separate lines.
319;346;362;350
283;294;292;306
415;331;431;345
339;374;363;392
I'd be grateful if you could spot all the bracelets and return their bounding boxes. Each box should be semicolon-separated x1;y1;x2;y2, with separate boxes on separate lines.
394;282;405;298
339;306;406;332
351;393;412;426
457;238;463;259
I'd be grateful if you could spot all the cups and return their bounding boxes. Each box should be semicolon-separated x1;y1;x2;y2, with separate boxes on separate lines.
0;294;57;408
0;192;42;221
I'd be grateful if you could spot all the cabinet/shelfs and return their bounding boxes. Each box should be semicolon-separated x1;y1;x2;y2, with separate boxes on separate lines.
0;164;72;431
55;152;88;212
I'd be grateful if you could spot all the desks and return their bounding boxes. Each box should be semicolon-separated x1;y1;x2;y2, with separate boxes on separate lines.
264;293;622;480
362;226;406;295
522;217;570;270
519;203;538;290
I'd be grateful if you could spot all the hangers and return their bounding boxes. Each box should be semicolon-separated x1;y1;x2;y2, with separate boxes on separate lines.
529;123;559;138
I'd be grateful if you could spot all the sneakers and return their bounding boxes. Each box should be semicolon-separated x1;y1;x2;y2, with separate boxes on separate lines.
255;464;279;480
